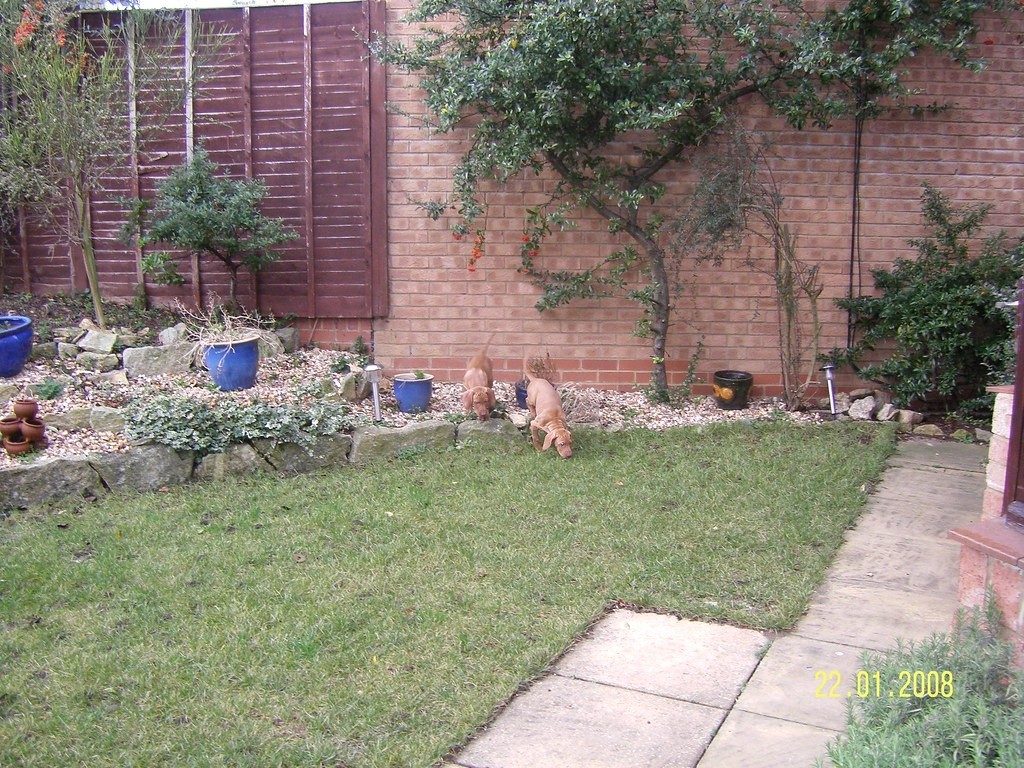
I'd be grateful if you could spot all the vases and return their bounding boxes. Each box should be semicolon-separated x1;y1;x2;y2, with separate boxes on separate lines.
0;399;49;455
713;370;753;410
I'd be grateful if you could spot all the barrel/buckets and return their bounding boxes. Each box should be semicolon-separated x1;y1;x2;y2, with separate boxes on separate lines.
714;370;753;410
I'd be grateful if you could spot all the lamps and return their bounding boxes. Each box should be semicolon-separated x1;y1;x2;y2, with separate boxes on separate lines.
819;364;835;380
365;365;383;383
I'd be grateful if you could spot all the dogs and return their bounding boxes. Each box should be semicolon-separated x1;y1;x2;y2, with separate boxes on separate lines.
523;353;573;459
460;333;496;422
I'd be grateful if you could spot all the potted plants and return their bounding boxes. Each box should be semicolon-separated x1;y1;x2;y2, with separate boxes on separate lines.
516;347;557;409
394;370;434;413
174;290;277;392
0;316;32;378
